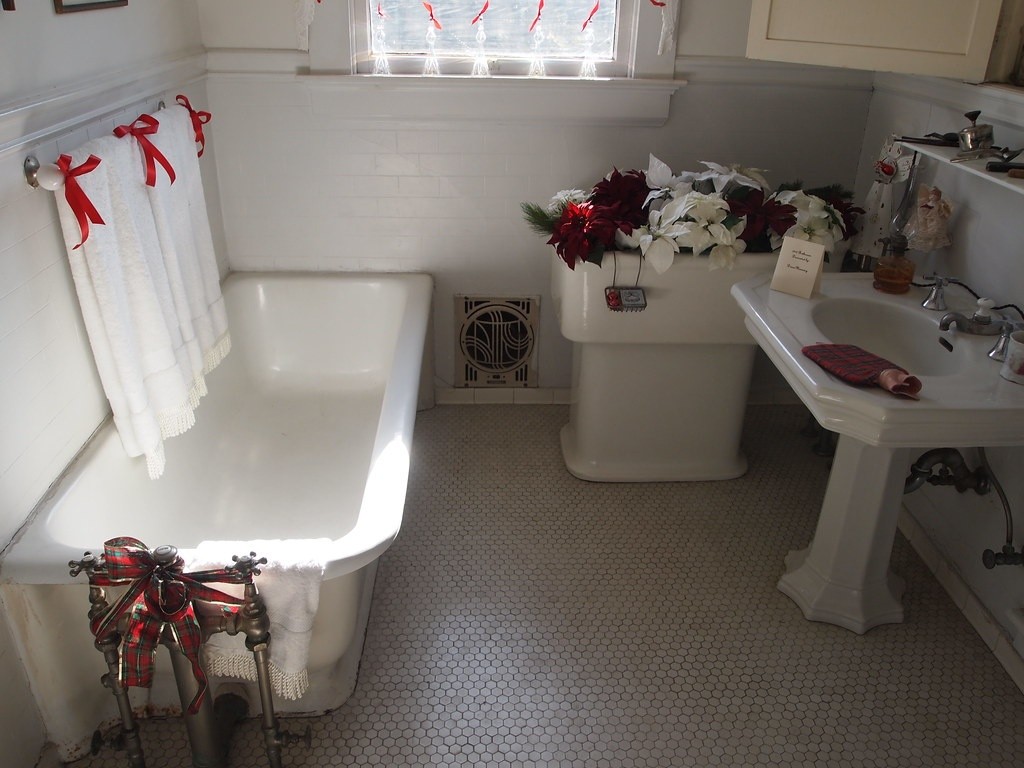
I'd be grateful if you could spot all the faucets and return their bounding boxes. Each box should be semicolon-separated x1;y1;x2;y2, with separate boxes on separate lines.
938;294;996;335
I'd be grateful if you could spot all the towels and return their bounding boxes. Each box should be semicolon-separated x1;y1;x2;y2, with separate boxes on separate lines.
195;538;328;703
51;102;234;481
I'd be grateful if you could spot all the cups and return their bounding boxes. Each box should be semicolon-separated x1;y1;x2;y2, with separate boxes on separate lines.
1001;331;1024;385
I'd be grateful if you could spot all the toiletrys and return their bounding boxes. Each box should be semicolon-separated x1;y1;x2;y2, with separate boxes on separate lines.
873;234;918;294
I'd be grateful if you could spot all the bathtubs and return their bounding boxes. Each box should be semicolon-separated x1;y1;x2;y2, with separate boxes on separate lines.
1;272;433;756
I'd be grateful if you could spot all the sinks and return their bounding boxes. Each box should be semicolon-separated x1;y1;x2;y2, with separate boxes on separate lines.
731;270;1019;445
